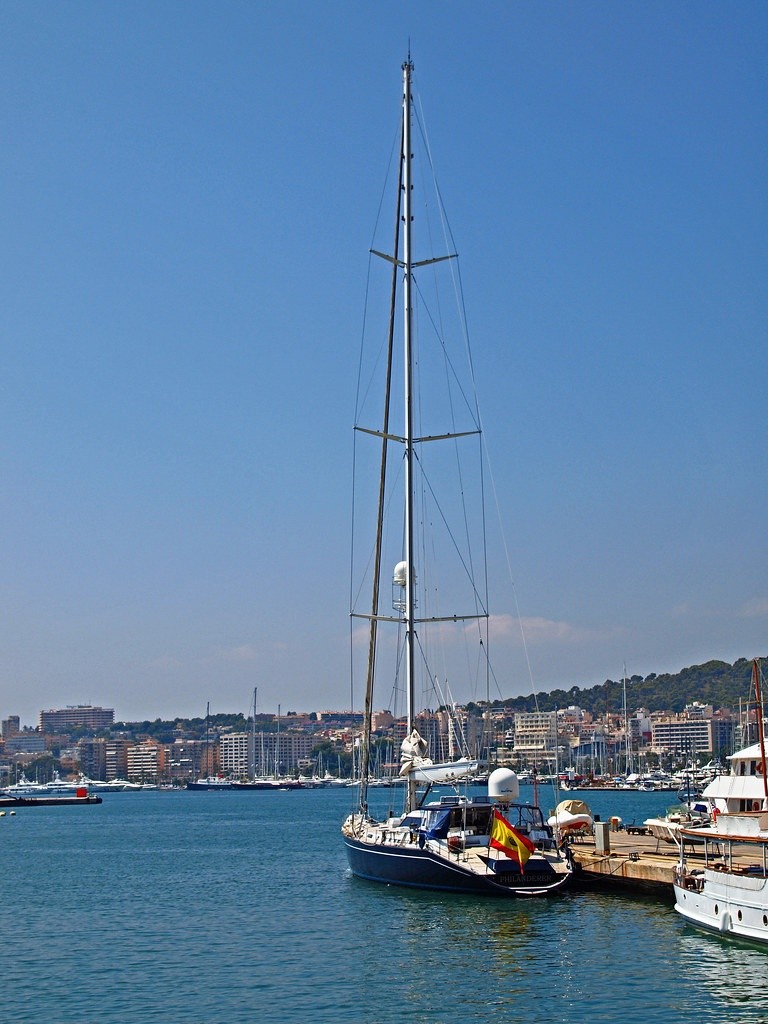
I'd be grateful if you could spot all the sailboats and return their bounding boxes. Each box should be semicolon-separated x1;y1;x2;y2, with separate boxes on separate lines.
523;664;765;842
1;680;521;806
321;40;584;902
660;657;768;946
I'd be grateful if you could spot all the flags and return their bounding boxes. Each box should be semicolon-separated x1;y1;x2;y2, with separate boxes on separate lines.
489;808;536;876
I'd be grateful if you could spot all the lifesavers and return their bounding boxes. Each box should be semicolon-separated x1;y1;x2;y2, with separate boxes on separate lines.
758;762;767;774
